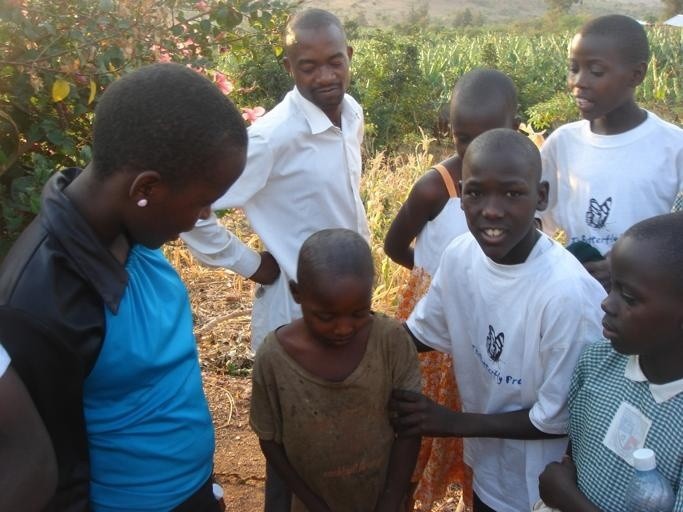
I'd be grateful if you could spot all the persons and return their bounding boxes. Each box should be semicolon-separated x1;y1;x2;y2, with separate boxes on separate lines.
177;8;370;511
247;230;420;512
0;61;248;512
538;209;683;512
384;68;532;512
533;12;682;294
0;342;59;512
386;129;611;512
